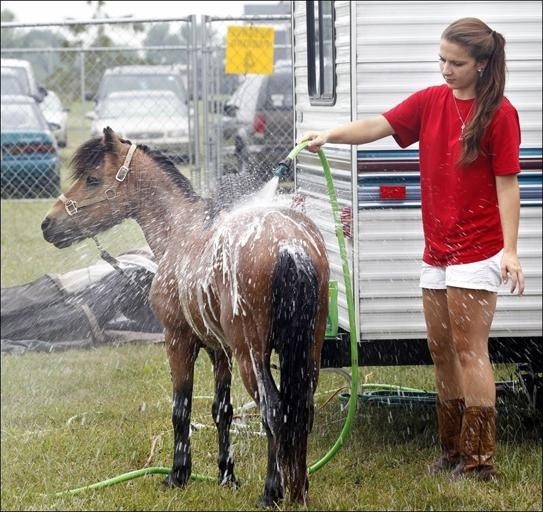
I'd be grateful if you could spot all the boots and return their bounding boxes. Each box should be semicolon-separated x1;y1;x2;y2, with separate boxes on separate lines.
449;406;501;481
422;396;465;474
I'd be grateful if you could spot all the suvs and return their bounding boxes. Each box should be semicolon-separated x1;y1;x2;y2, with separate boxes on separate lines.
83;63;202;109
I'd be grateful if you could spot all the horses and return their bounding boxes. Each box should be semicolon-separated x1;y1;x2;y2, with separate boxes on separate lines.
38;123;333;509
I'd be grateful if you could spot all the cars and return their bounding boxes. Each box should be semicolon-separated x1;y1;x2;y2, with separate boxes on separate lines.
207;58;294;183
0;59;71;197
84;90;198;166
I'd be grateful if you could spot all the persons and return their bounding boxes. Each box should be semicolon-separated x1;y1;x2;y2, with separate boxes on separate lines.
297;13;527;485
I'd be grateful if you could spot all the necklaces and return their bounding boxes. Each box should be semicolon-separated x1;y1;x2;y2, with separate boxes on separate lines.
451;90;478;142
